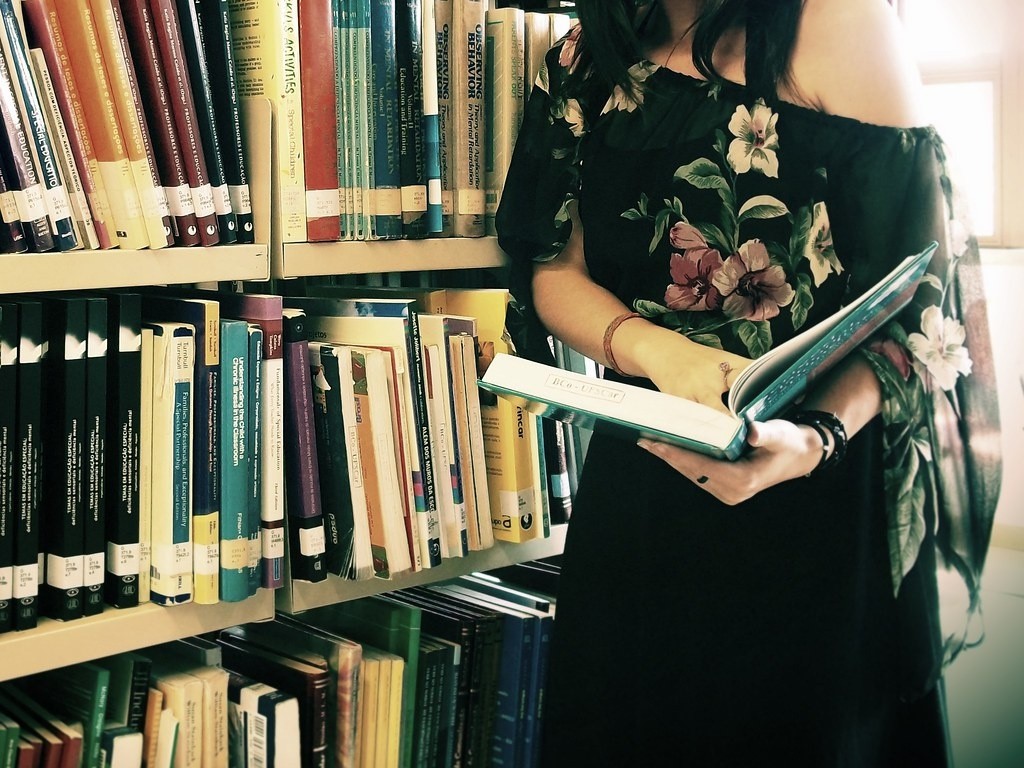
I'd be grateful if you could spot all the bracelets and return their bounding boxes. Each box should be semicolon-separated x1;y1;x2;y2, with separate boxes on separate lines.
786;407;848;480
603;313;647;378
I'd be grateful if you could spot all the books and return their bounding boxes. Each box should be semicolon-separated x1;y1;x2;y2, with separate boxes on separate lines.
0;0;607;766
475;238;941;464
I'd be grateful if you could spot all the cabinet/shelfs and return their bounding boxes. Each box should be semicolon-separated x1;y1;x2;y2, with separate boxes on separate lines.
0;231;577;768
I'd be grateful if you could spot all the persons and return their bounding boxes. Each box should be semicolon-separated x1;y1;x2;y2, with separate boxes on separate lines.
497;1;1003;766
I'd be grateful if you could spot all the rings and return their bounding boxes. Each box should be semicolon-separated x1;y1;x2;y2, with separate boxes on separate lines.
697;474;709;485
719;362;736;391
